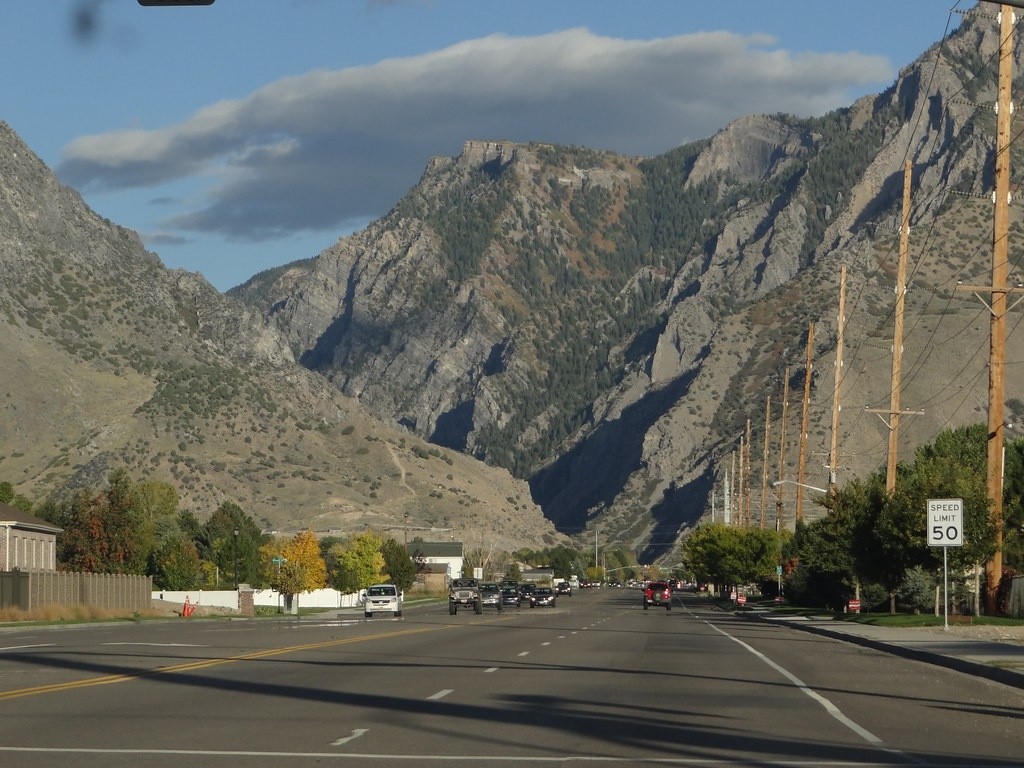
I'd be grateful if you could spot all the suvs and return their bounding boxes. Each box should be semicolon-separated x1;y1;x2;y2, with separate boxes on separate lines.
519;584;537;601
555;582;571;597
643;581;672;610
448;578;484;615
480;581;502;610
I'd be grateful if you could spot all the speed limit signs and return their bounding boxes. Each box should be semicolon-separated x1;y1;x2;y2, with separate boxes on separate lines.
926;499;963;546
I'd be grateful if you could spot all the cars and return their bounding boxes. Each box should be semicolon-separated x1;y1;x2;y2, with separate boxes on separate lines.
581;580;591;588
529;588;556;607
363;584;404;617
593;578;601;587
609;579;617;588
628;580;645;587
499;581;521;608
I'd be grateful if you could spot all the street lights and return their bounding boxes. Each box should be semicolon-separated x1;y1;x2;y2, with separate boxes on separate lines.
772;480;834;516
273;560;281;613
234;527;239;590
